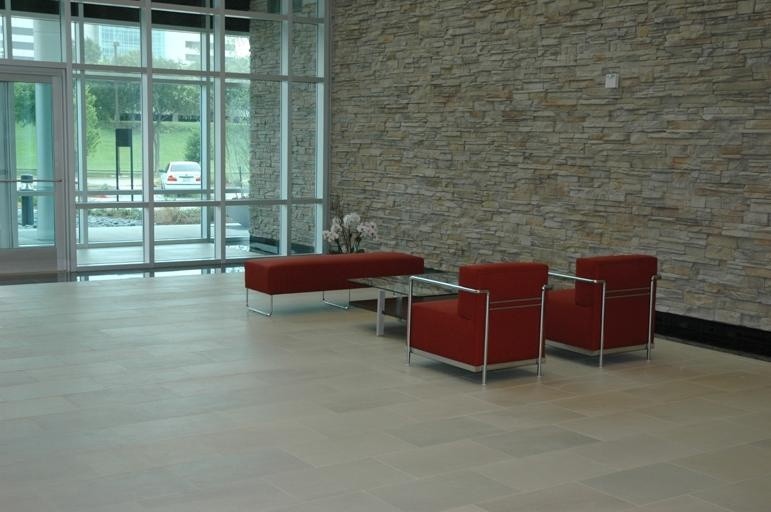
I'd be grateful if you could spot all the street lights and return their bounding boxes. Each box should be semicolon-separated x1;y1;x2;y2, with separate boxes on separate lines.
113;41;122;122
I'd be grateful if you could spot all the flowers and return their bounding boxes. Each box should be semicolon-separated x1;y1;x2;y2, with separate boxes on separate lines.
321;211;380;251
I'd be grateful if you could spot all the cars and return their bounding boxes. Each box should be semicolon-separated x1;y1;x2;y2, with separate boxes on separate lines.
158;161;202;192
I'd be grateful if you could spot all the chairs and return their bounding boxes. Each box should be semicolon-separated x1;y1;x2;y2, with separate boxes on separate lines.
542;252;663;370
404;260;554;386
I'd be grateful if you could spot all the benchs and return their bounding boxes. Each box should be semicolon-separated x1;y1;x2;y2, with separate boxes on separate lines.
243;249;426;321
